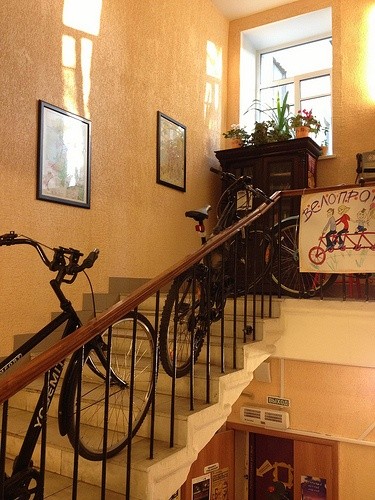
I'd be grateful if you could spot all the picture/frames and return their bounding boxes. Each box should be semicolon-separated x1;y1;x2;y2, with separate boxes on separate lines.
156;111;186;192
36;99;91;209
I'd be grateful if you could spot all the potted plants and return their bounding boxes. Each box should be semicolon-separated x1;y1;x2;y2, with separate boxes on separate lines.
247;92;294;144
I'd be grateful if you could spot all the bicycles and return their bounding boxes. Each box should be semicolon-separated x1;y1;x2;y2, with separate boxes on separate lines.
0;228;159;500
158;167;340;379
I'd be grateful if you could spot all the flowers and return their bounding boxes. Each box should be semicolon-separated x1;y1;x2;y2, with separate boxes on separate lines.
291;109;320;133
223;124;250;141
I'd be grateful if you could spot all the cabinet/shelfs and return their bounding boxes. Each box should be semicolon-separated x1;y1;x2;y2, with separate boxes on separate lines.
214;137;322;293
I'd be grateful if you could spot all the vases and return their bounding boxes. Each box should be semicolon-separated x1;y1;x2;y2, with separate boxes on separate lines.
295;126;311;138
231;139;243;148
